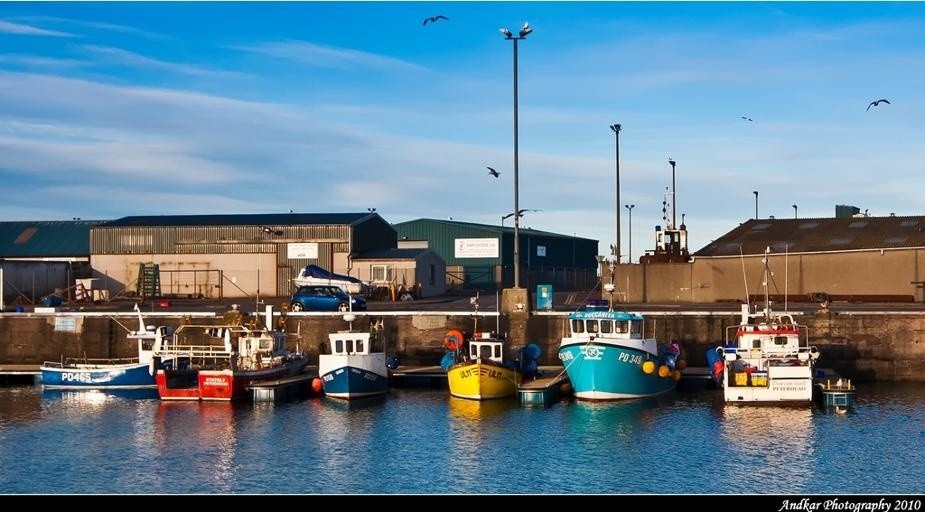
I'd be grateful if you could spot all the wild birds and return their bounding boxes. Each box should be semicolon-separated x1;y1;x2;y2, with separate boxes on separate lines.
741;117;752;121
487;167;501;177
423;16;448;26
866;99;891;111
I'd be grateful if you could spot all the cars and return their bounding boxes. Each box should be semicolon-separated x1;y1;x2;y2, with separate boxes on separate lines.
290;285;367;312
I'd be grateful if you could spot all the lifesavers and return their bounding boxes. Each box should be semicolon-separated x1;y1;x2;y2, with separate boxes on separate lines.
445;330;465;351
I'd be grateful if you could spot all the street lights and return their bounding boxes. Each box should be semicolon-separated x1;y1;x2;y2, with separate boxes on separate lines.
669;161;676;229
499;21;532;288
610;124;635;264
753;191;758;219
793;205;797;219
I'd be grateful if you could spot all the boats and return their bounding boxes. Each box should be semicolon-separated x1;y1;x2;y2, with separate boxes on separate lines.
319;282;390;400
34;270;310;401
447;291;523;401
558;242;687;401
293;265;367;293
707;250;821;402
